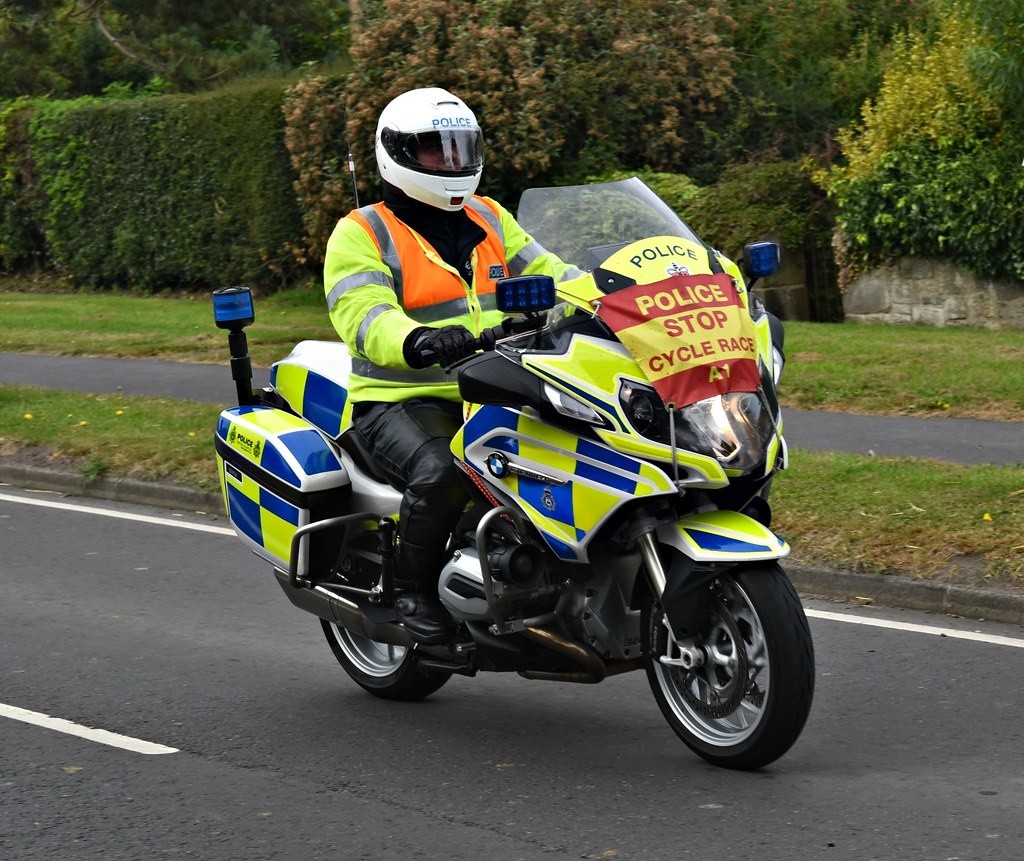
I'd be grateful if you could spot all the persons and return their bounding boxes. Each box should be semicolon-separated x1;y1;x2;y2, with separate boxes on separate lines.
324;88;592;644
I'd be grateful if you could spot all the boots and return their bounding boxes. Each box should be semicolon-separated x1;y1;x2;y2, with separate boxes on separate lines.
390;539;458;645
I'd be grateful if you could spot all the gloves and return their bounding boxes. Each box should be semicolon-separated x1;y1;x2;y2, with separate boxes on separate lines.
413;325;475;374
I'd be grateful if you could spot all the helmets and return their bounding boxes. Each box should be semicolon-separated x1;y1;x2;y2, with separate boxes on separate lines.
374;87;485;212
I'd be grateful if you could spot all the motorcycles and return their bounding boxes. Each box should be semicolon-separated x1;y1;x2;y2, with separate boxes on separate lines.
211;178;815;770
